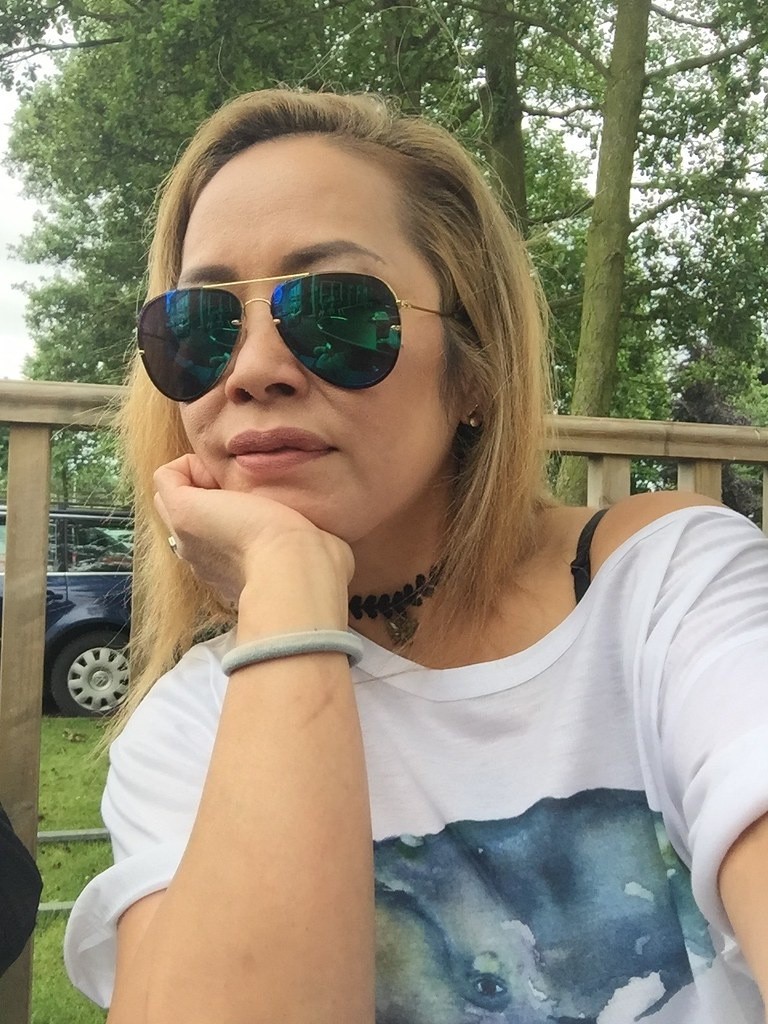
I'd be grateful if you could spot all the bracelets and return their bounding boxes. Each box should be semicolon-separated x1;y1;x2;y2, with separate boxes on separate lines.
220;629;364;678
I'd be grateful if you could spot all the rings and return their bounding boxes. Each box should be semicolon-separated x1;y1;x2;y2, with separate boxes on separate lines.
168;536;177;554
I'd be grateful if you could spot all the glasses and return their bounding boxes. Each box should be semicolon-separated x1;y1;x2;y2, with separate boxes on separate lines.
137;272;454;402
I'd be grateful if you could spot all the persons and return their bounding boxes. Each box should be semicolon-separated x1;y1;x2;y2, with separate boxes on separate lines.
63;88;768;1024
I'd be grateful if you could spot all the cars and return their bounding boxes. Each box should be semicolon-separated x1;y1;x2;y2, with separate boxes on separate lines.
0;504;134;715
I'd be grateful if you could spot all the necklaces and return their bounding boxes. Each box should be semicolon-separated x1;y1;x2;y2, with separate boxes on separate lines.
348;545;466;647
